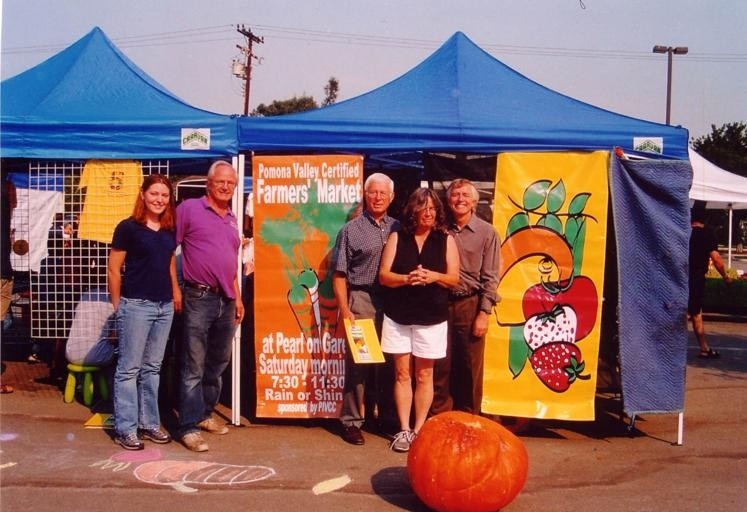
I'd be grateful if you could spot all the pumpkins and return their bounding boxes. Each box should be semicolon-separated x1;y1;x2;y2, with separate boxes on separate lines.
406;411;531;512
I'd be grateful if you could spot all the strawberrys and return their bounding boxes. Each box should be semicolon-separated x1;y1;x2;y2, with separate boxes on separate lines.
523;305;577;351
529;341;591;393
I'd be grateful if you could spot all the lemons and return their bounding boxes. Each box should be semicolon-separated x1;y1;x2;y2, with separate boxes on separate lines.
494;254;559;326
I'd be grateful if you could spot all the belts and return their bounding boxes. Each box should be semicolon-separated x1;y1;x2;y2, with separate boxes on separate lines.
185;282;222;294
451;290;478;301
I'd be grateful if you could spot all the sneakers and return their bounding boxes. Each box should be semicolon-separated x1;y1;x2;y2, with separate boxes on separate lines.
114;433;141;451
182;432;208;452
389;430;411;453
28;354;40;363
195;418;229;435
138;428;171;444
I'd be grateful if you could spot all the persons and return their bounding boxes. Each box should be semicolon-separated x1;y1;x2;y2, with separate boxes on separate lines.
428;178;501;420
689;206;734;360
378;189;461;454
331;171;402;446
1;160;253;453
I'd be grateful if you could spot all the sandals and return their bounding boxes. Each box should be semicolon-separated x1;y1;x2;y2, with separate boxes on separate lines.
702;348;719;357
0;385;13;393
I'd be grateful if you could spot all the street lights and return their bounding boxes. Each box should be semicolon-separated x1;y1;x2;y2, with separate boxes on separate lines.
652;45;689;127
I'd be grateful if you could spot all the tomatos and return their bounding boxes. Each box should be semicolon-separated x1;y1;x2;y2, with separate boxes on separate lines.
522;269;598;341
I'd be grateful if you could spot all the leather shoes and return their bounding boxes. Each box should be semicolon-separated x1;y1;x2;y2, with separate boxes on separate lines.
343;426;365;445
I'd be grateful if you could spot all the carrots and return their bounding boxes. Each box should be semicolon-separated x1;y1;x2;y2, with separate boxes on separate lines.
288;271;346;388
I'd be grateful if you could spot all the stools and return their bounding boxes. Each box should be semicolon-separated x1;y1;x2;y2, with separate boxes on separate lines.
64;364;108;406
